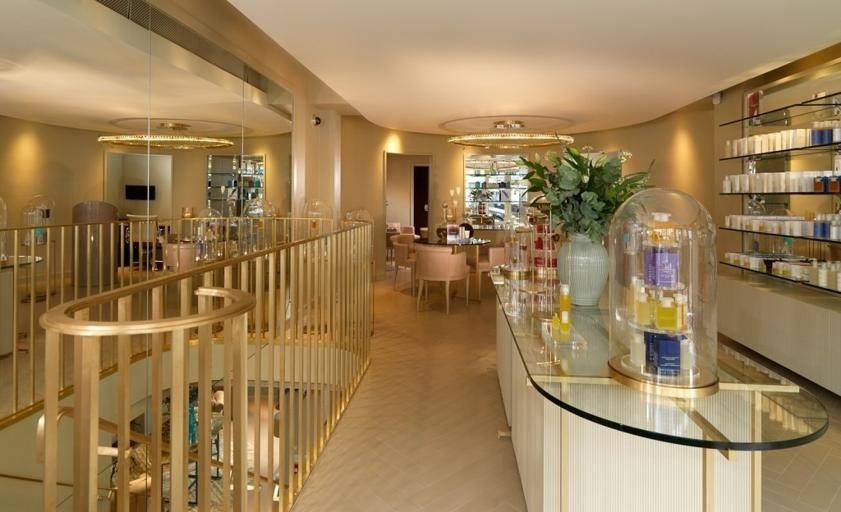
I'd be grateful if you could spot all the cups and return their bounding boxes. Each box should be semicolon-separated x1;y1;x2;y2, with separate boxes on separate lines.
464;230;470;239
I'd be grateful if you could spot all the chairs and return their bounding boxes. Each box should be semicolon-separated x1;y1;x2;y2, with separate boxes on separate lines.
126;213;163;272
382;222;505;314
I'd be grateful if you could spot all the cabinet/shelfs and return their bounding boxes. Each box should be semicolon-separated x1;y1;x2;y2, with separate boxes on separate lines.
465;171;528;231
715;91;840;301
211;169;266;202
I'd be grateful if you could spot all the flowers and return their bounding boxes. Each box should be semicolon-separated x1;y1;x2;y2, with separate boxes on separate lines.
508;129;658;243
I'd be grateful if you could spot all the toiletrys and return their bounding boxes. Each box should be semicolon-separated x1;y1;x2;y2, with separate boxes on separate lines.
552;284;571;342
625;213;694;376
722;119;841;292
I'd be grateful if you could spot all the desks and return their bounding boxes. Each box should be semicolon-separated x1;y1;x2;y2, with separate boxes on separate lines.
0;254;44;359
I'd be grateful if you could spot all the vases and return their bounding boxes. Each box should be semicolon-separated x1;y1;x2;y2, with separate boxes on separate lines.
557;230;611;307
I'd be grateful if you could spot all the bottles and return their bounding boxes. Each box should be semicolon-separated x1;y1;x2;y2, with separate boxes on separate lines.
436;202;451;239
552;284;573;344
722;124;841;289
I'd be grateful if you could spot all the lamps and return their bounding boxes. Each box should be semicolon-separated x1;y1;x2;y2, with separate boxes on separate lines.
446;121;575;151
97;124;234;153
